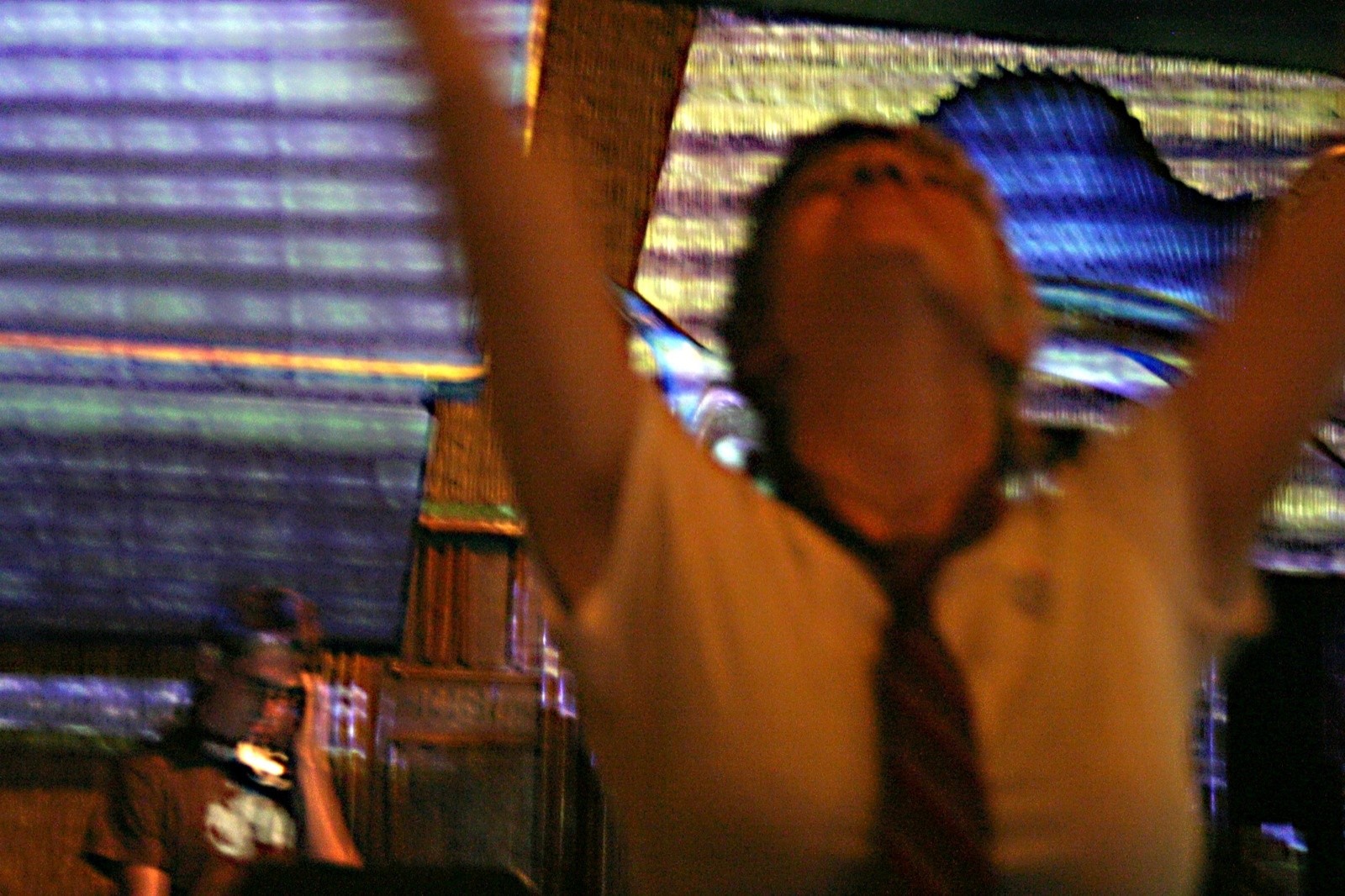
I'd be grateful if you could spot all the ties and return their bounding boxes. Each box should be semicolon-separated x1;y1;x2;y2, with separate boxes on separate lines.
771;472;1006;896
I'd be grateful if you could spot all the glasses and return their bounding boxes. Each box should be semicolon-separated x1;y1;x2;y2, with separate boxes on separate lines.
217;664;308;718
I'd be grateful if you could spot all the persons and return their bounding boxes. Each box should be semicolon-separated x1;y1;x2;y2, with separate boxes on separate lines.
369;0;1344;896
79;583;368;896
